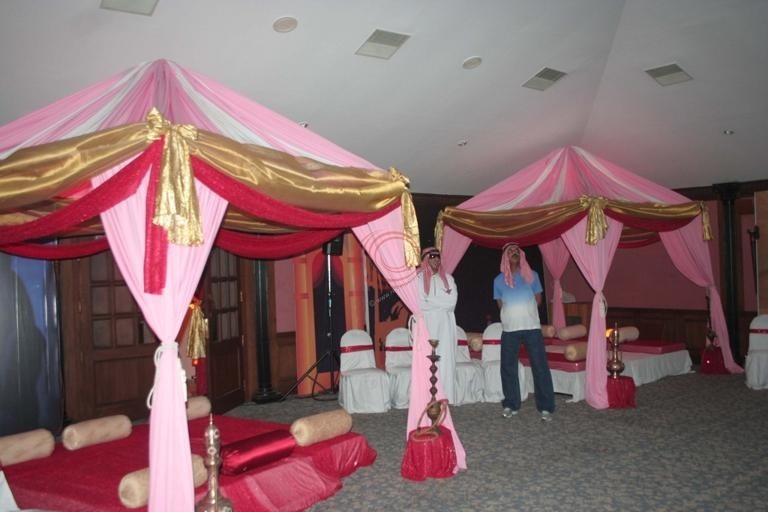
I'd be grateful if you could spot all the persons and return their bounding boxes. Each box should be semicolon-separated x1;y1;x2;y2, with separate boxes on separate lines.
413;243;458;409
490;239;554;423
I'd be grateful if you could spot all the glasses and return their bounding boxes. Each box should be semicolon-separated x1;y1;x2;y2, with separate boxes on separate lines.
429;253;440;259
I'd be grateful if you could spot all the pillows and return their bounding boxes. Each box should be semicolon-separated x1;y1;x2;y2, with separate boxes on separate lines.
557;323;587;340
467;332;482;352
116;395;354;508
564;341;587;361
541;324;556;338
62;413;132;451
610;326;640;345
0;428;55;468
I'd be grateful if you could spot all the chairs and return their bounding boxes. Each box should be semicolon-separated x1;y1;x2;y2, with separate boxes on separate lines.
337;329;392;414
745;313;768;390
482;323;528;405
455;324;483;404
385;327;414;410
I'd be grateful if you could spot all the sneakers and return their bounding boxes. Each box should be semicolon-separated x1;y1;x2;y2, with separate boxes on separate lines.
541;411;549;420
502;408;517;418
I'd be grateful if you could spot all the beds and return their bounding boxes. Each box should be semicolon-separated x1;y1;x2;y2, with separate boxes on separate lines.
0;414;376;512
466;324;693;403
